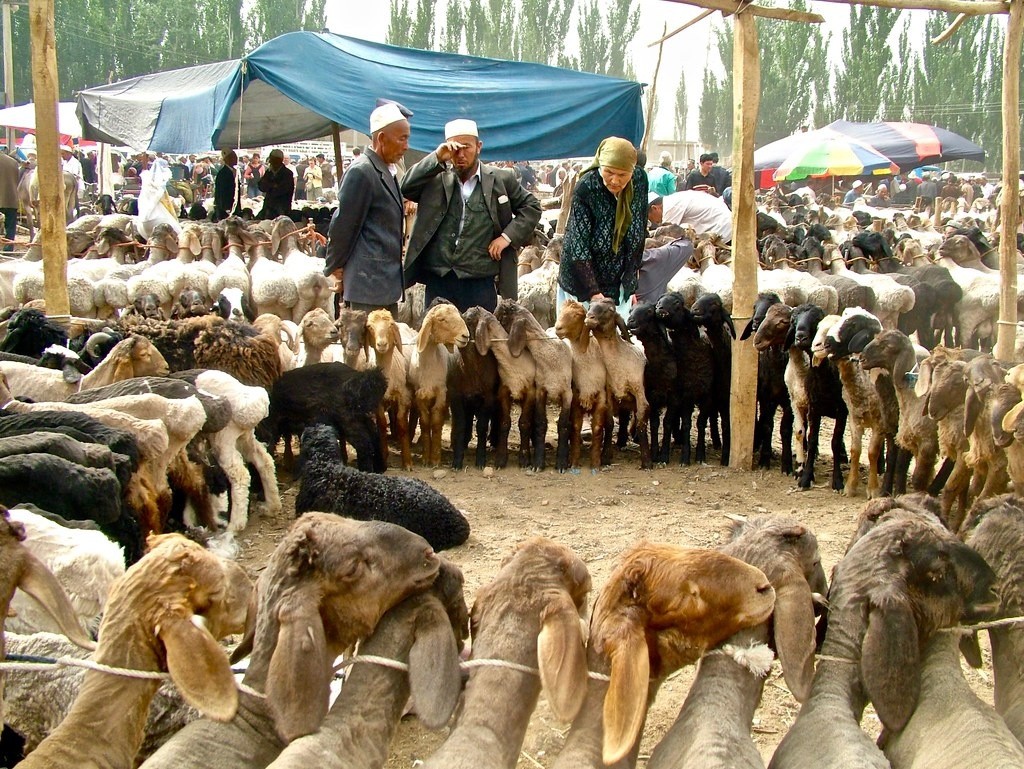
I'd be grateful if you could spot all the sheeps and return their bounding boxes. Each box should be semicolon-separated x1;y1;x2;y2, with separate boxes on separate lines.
0;212;1024;542
0;503;1024;769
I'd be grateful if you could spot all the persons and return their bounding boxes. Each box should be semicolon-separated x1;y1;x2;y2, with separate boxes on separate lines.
398;117;543;319
21;142;359;216
324;102;411;331
553;136;648;332
487;151;735;209
772;166;1024;209
635;235;696;311
644;188;735;251
0;145;21;253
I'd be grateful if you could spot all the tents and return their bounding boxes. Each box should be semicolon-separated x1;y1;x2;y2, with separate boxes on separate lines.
73;27;645;160
0;99;103;146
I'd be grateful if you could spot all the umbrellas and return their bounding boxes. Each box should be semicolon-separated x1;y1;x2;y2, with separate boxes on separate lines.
748;117;987;190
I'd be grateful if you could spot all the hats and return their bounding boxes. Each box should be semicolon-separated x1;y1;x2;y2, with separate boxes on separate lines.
943;220;962;229
877;184;887;190
26;149;37;157
370;103;406;134
852;180;862;188
60;146;73;153
270;149;284;159
129;167;137;174
221;147;233;158
444;118;479;141
300;155;307;161
658;151;672;167
